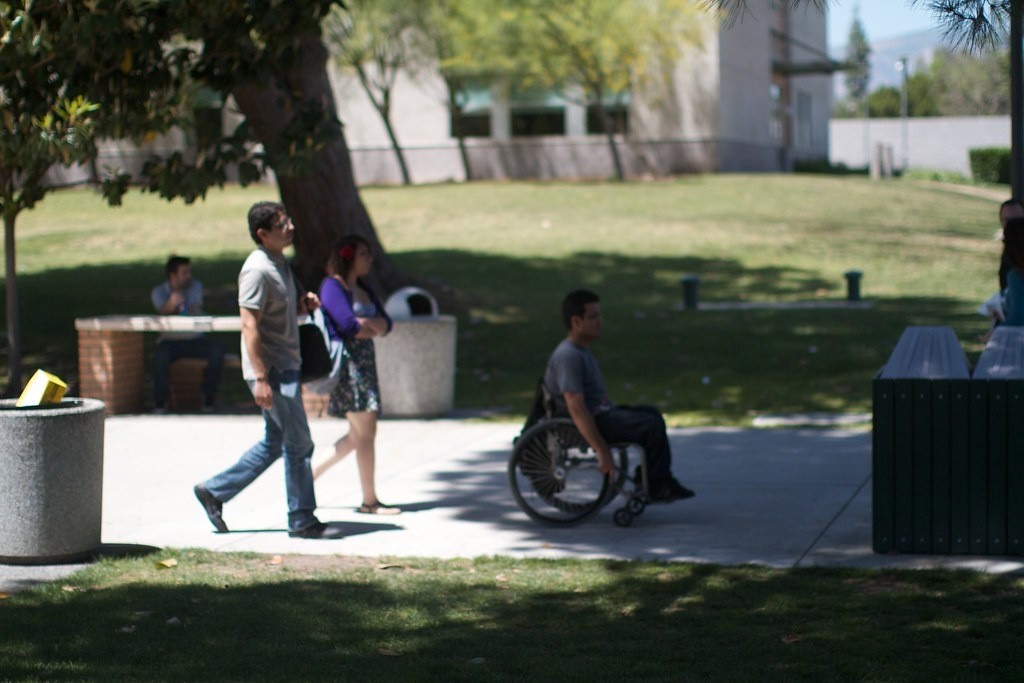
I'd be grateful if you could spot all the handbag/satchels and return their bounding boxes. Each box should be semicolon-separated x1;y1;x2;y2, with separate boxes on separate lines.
296;324;333;381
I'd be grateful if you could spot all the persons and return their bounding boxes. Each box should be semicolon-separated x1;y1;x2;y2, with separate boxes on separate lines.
195;201;345;537
314;231;405;517
975;198;1024;342
546;289;695;503
151;254;224;414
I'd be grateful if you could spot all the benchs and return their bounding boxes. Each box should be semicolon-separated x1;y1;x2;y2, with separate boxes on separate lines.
165;356;243;395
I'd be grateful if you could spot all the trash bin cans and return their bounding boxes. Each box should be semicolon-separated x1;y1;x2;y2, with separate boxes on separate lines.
372;287;459;419
0;397;108;565
871;325;1024;559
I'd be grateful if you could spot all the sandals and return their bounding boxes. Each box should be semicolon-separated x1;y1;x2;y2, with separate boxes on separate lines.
357;501;401;514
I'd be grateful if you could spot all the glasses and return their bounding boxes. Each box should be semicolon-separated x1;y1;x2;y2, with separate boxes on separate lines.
270;214;289;230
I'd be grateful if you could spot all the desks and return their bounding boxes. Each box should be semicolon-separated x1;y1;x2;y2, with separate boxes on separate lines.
76;314;329;417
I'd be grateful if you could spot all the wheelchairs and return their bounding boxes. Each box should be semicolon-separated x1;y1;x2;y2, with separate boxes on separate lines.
507;375;663;529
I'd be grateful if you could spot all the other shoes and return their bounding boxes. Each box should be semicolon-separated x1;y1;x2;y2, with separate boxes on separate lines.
200;392;215;413
194;483;229;532
646;480;695;502
289;519;344;539
156;402;168;414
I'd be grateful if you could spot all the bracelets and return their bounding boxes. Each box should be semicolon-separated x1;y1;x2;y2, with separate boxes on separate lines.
253;376;269;381
